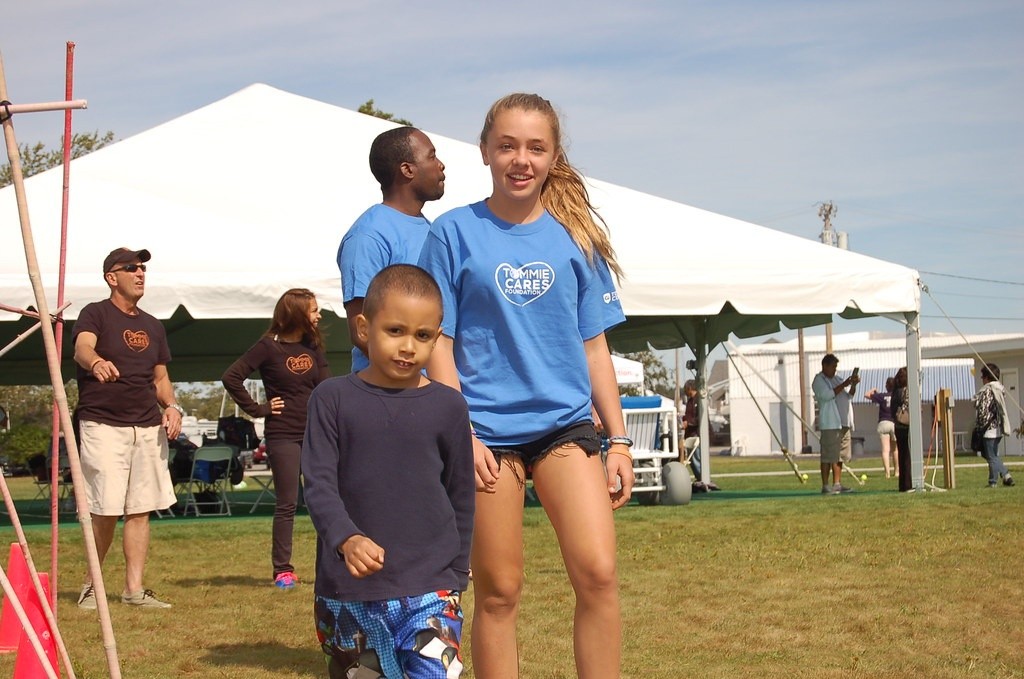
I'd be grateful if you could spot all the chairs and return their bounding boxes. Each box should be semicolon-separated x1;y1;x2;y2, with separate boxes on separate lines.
669;436;700;465
250;474;276;512
26;446;234;518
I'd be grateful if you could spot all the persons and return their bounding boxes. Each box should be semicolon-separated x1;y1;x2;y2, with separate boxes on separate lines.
891;367;910;491
223;289;329;588
865;378;899;476
972;363;1014;487
300;263;473;679
72;248;182;611
815;353;861;495
332;125;447;375
418;93;632;679
680;381;701;480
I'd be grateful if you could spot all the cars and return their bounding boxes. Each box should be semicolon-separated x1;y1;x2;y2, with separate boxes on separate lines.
709;413;731;445
237;439;253;468
253;436;268;464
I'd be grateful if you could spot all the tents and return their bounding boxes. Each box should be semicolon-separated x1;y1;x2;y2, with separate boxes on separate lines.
611;354;644;383
0;84;927;488
726;333;1024;456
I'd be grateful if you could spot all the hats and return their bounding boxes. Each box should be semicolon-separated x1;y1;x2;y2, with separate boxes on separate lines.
103;247;151;273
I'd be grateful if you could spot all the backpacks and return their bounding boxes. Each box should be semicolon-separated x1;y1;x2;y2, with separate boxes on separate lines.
895;387;909;424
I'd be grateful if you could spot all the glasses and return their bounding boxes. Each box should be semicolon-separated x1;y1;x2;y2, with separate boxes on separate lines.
111;263;146;272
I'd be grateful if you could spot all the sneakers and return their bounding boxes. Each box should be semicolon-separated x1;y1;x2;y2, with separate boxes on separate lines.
77;583;97;609
274;571;298;589
121;588;172;609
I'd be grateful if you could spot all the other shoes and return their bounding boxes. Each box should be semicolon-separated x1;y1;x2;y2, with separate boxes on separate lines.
1003;476;1015;486
821;487;840;494
831;485;854;492
985;481;997;487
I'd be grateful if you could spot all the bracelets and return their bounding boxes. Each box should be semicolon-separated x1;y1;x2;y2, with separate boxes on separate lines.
92;360;100;369
170;403;183;417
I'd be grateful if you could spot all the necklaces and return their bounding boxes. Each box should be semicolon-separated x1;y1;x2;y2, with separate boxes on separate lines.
489;196;542;224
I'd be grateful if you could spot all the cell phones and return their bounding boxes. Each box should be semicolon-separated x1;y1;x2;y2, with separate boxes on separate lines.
852;368;859;376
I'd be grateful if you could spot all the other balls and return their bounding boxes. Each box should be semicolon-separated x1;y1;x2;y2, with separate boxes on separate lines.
803;474;808;480
861;474;867;481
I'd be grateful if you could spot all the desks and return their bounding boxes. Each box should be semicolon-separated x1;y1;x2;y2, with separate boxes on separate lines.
953;431;969;452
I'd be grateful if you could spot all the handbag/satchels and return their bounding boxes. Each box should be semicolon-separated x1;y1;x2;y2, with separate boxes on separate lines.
217;413;262;449
194;489;220;514
971;427;984;450
173;447;244;484
